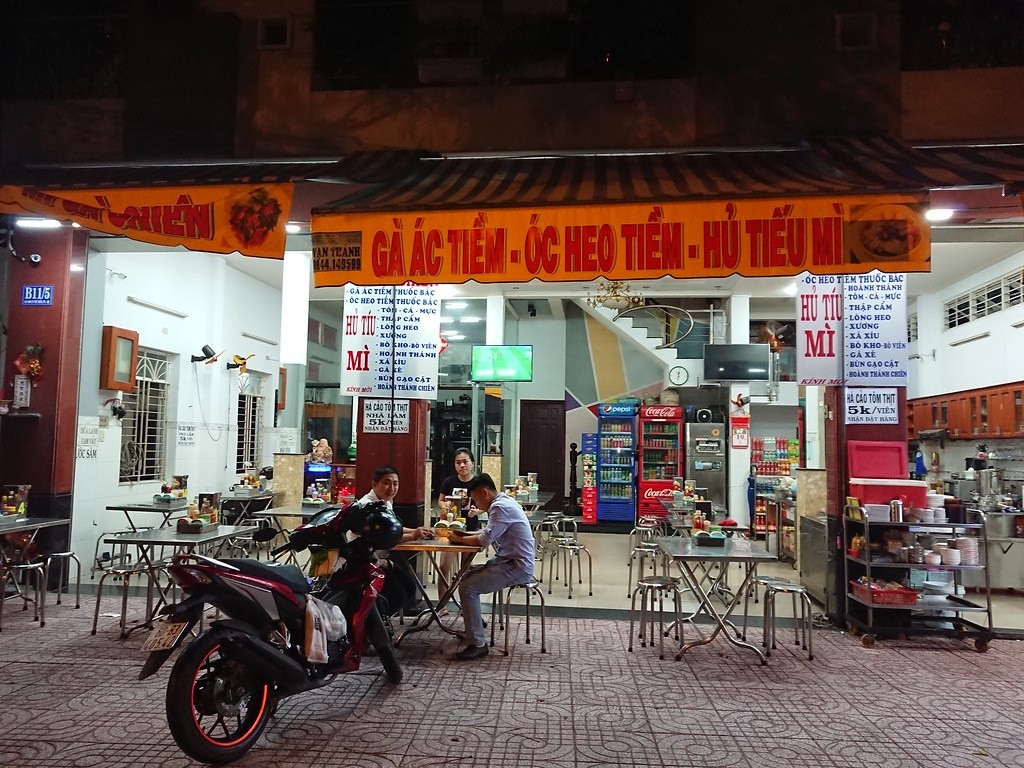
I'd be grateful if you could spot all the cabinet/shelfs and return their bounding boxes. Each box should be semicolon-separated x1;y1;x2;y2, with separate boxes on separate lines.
749;448;800;561
906;382;1024;439
839;506;994;652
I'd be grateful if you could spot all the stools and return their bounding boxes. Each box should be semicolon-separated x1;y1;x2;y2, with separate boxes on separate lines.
627;515;814;661
1;508;591;658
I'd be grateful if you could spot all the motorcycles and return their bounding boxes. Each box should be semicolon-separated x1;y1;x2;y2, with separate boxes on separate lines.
137;496;412;765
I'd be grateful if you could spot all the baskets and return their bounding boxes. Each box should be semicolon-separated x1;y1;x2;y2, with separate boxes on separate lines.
850;579;919;606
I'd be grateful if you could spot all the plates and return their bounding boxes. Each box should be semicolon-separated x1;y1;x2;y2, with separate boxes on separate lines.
927;495;944;507
922;581;950;590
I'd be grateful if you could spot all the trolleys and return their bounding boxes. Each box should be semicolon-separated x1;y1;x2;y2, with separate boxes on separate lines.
841;503;995;652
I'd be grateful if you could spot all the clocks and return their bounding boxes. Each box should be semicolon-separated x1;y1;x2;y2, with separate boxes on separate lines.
668;366;689;385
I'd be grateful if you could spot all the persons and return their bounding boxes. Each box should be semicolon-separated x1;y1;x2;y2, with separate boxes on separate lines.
455;473;534;662
316;438;333;463
438;448;484;617
346;466;435;565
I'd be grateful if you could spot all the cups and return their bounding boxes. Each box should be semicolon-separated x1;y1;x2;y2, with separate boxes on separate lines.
891;500;902;522
934;508;945;523
922;509;934;524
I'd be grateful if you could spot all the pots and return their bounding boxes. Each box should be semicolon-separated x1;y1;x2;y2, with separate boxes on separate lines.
978;468;1005;495
979;514;1016;538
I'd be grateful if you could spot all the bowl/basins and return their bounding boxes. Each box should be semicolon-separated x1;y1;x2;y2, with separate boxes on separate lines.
925;536;979;566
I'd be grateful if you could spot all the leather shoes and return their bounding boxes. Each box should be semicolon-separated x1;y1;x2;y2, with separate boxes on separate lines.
456;643;489;660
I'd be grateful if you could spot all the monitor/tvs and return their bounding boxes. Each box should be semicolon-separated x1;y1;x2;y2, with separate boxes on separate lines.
702;344;770;381
471;345;534;383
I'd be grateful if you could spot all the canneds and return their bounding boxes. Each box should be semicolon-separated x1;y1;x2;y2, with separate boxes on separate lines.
643;424;679;448
600;450;630;464
601;423;631;432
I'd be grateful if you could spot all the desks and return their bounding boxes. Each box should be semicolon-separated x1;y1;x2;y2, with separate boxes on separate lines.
653;536;778;666
653;495;727;538
660;514;749;569
1;491;571;646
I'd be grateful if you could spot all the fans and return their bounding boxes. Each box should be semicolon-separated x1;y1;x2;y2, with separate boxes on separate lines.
192;345;256;376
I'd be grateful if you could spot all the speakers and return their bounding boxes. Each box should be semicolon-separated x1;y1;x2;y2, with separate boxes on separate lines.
696;409;712;423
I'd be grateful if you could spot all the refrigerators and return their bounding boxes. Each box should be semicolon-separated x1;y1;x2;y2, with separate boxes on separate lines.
596;403;635;520
685;423;726;516
638;406;682;525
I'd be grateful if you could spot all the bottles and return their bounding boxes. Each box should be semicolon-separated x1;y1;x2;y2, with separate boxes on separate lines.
162;479;183;498
306;483;328;503
643;423;677;480
693;510;709;532
187;499;217;522
240;475;254;485
752;437;799;533
601;424;633;497
441;501;457;523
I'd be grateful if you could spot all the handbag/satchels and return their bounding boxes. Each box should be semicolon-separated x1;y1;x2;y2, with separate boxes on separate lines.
304;596;328;664
312;595;347;641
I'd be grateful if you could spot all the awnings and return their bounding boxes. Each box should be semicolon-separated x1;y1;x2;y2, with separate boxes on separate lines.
0;163;332;261
310;154;932;287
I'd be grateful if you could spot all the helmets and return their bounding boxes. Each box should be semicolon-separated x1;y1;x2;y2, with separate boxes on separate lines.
362;511;403;551
